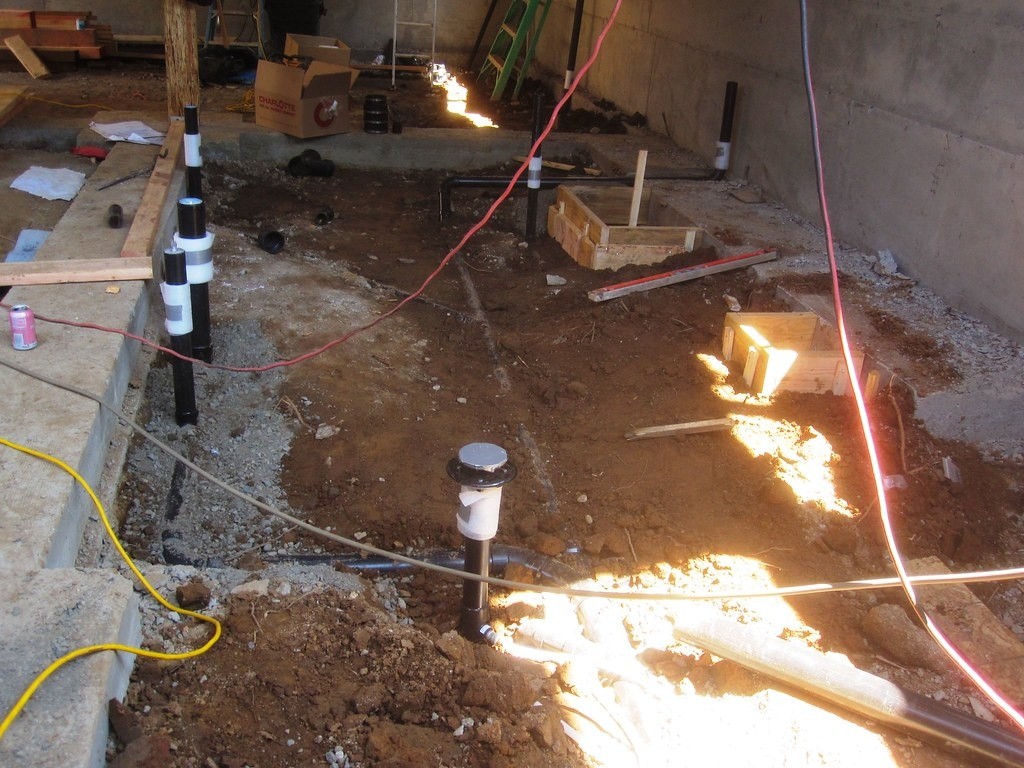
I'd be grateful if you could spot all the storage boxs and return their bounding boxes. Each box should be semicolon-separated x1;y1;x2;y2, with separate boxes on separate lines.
253;55;361;141
282;33;351;73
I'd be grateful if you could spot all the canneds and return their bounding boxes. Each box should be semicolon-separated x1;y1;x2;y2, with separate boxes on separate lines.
7;304;38;350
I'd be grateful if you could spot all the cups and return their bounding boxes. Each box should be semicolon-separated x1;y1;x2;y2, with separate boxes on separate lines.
76;19;85;29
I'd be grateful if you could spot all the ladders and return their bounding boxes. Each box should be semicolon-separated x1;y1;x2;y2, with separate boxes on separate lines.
477;0;556;104
391;0;439;97
203;0;266;68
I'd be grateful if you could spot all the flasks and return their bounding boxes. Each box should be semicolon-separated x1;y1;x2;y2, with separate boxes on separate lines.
363;94;389;133
